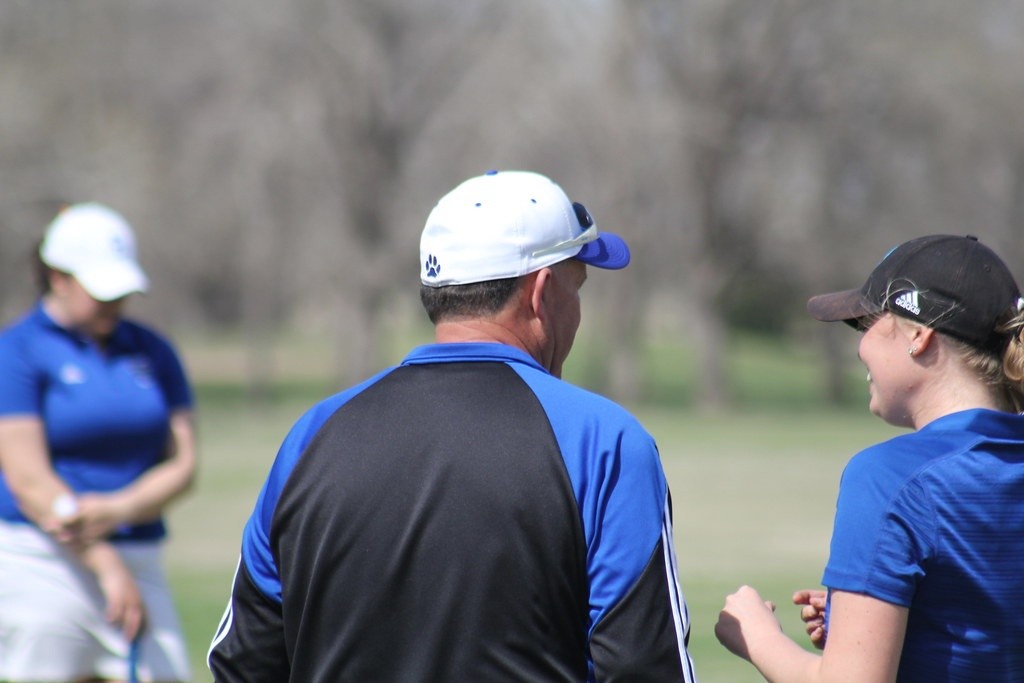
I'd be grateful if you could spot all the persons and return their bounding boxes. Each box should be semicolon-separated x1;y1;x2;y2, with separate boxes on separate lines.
710;234;1023;683
205;170;696;683
1;201;200;681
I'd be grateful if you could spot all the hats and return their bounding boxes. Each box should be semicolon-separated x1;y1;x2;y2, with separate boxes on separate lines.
420;171;630;286
39;201;150;300
809;233;1021;352
